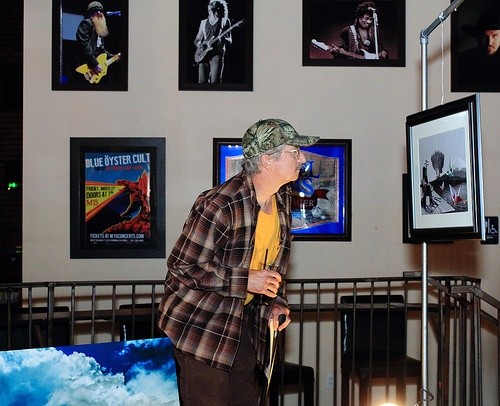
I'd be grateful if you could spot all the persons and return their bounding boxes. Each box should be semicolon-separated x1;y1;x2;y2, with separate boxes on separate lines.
460;14;500;86
194;0;232;84
158;119;320;406
77;1;121;75
330;2;389;60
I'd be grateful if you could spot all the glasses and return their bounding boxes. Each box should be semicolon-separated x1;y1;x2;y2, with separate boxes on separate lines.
91;9;103;12
273;148;300;159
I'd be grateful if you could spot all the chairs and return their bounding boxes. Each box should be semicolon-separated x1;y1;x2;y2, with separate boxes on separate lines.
268;361;314;406
119;303;162;342
0;306;71;351
340;295;423;406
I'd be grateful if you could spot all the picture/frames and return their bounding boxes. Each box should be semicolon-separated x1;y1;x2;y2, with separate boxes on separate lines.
303;0;405;66
213;138;352;241
52;0;129;91
403;93;485;244
178;0;253;91
70;137;166;260
450;0;500;92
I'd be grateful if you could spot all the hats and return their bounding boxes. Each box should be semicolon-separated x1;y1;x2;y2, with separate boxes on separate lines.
86;1;103;11
241;119;320;158
479;15;500;30
